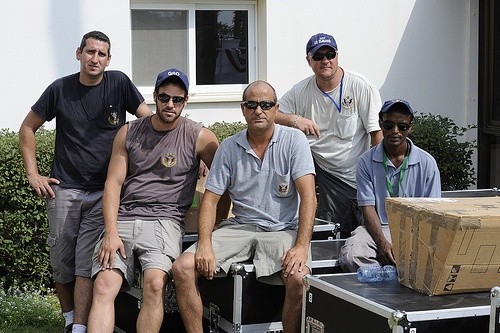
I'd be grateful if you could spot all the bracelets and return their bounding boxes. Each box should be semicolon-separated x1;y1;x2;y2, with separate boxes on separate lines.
292;115;301;127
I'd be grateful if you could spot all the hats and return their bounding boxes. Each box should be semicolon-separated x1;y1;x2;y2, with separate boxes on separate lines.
307;33;337;55
156;68;189;95
380;100;414;123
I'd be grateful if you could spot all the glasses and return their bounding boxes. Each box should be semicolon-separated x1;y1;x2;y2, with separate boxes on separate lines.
242;100;276;110
156;92;187;103
380;119;411;131
312;50;336;61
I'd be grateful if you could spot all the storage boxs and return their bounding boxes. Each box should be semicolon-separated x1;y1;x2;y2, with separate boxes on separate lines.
114;188;500;333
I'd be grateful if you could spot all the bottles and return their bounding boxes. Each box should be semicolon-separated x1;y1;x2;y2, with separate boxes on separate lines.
356;265;398;282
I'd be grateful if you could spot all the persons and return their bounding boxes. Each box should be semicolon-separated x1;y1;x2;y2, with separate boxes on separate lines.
338;99;441;272
87;69;231;333
172;80;317;333
274;32;384;239
19;31;154;333
